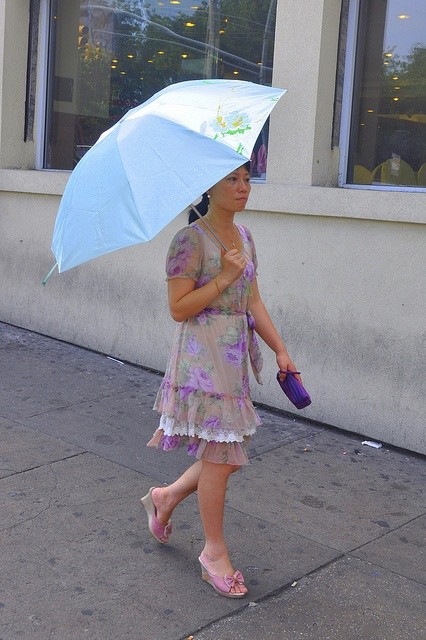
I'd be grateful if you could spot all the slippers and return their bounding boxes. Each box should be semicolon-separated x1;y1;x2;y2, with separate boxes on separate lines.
140;486;173;544
198;556;246;599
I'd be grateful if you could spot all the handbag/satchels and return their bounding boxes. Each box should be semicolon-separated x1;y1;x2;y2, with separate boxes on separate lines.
276;370;312;409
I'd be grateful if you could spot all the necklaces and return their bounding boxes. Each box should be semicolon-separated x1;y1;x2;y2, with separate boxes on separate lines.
224;235;238;247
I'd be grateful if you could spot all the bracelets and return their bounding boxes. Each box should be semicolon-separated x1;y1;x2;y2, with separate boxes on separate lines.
212;277;222;295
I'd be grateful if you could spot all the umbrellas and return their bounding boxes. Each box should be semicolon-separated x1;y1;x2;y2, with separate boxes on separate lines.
49;78;287;273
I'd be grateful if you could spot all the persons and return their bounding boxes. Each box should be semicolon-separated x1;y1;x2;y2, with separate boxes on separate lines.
140;161;303;598
